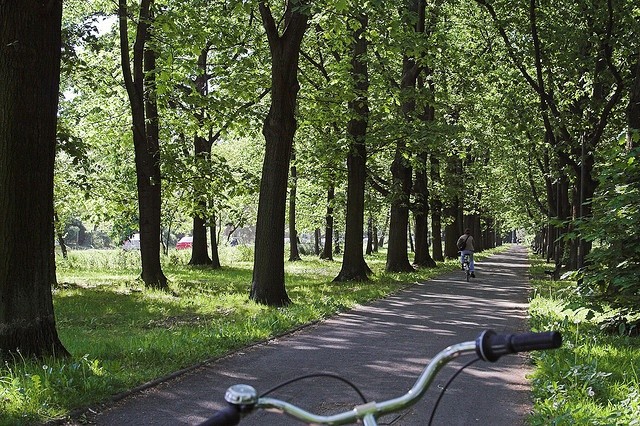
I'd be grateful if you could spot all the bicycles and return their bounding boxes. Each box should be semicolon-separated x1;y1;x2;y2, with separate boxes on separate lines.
542;270;572;280
457;251;476;282
195;329;562;426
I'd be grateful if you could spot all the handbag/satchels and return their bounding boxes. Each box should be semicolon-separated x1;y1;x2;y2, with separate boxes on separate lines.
457;241;466;251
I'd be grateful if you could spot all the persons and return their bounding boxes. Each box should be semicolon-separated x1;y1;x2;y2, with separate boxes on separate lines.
457;229;475;278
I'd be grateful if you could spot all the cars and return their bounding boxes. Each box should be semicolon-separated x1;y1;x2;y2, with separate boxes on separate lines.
124;233;141;251
176;236;193;249
